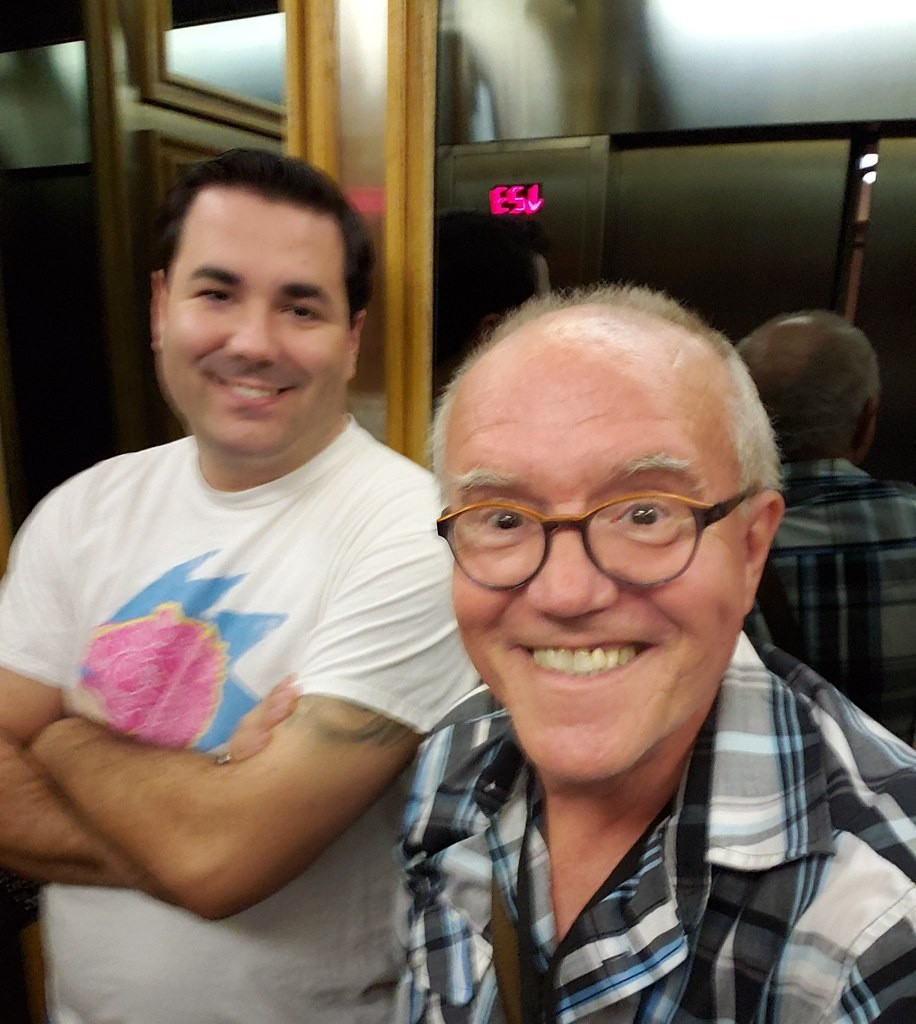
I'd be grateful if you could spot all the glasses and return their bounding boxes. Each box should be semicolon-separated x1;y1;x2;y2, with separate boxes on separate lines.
433;483;752;592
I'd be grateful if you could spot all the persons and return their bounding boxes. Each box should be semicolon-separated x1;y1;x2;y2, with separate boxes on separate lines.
729;309;916;756
396;283;916;1022
1;144;483;1024
